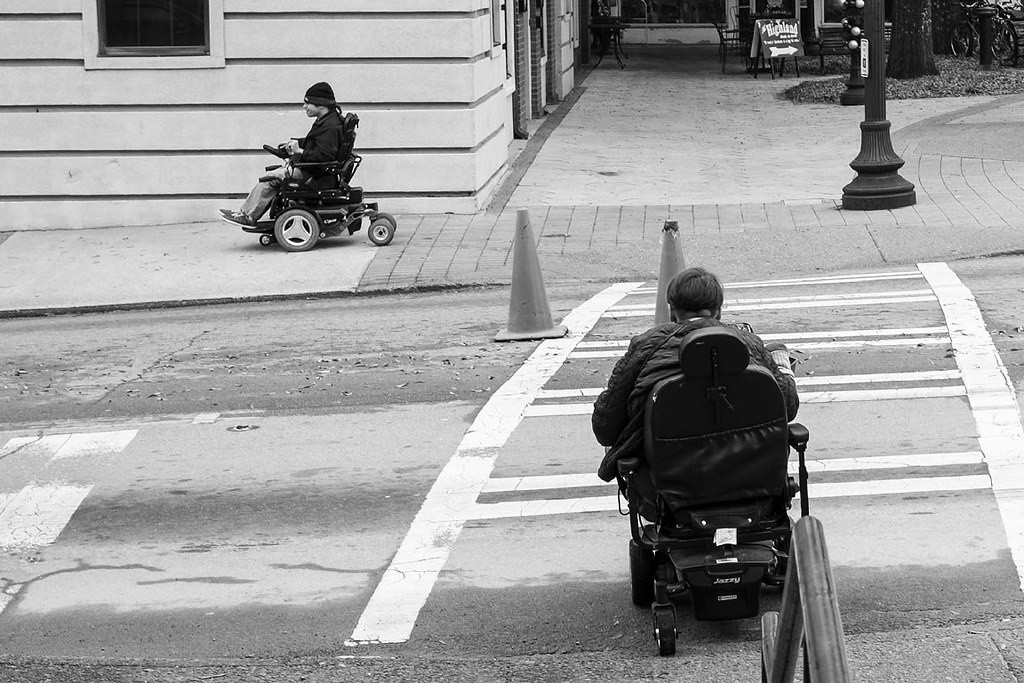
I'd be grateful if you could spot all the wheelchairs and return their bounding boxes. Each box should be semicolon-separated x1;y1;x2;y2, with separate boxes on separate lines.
617;326;809;655
242;113;397;252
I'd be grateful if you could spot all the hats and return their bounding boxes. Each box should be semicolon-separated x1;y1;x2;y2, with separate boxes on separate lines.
305;82;336;105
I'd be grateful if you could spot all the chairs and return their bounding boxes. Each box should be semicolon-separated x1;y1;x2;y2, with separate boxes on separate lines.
710;17;751;73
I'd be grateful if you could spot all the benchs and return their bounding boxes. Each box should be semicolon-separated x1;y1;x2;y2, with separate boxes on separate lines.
818;25;892;76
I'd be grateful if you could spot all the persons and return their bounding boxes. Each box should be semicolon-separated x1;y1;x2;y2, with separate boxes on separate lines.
592;268;799;447
218;82;342;228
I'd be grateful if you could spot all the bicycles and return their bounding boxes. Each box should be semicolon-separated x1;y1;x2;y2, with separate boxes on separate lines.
949;0;1018;60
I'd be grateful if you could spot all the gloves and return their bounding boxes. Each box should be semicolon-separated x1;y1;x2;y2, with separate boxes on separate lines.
764;341;791;369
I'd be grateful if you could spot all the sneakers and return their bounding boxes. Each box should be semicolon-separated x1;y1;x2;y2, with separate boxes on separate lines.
220;213;257;229
219;208;244;216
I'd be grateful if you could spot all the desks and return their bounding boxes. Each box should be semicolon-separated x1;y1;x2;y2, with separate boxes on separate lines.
736;13;796;74
588;23;630;69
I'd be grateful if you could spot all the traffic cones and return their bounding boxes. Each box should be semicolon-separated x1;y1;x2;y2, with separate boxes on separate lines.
493;208;568;341
652;220;686;325
